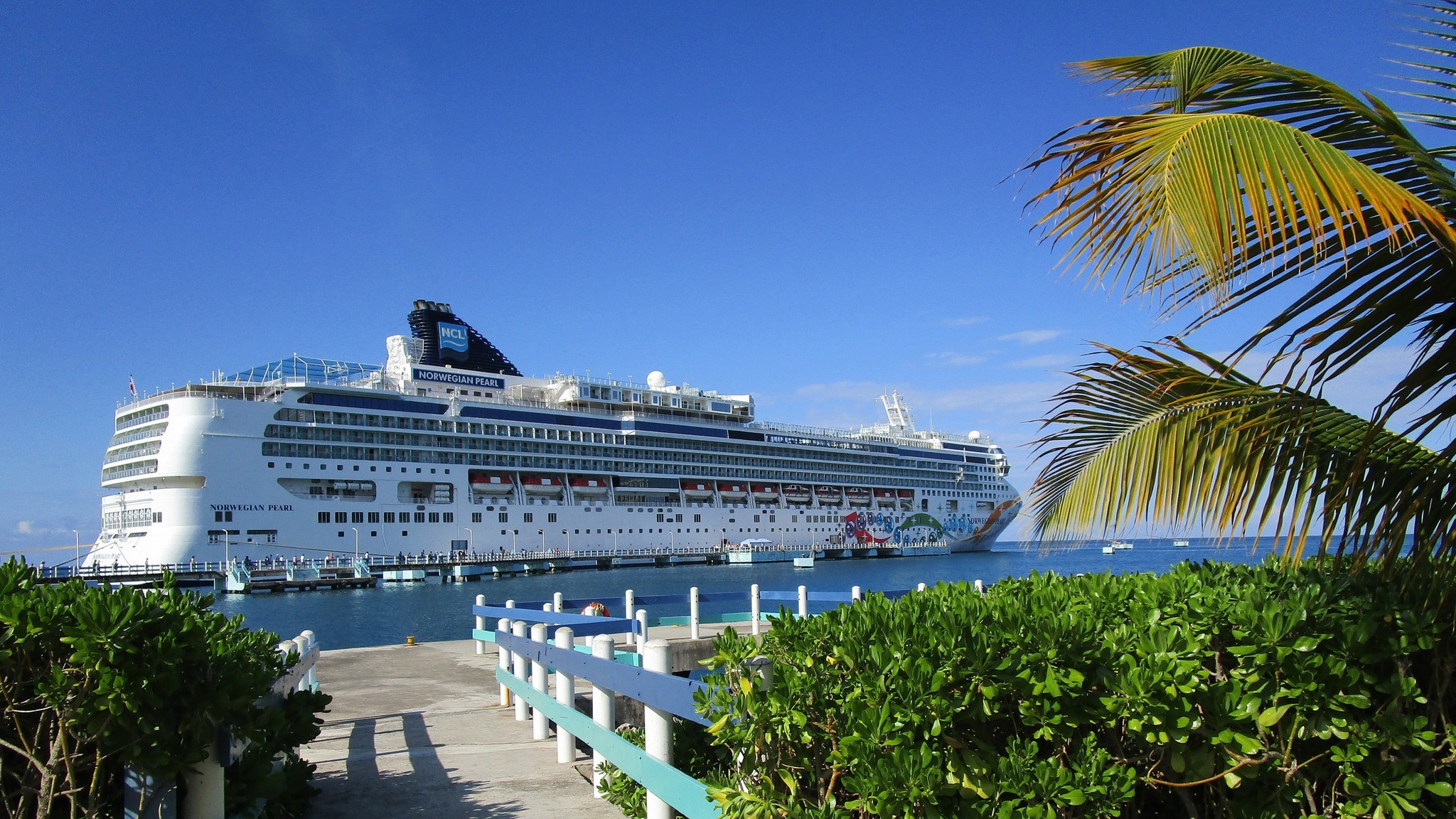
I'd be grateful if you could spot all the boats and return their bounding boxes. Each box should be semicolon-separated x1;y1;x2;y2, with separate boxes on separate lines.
78;299;1026;567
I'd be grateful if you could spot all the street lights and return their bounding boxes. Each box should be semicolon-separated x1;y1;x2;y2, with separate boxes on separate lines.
222;529;228;570
464;528;472;555
778;530;783;550
669;532;673;553
838;530;843;548
612;531;616;552
73;530;79;574
563;530;569;552
720;530;724;552
809;530;814;550
507;529;515;554
897;529;902;547
870;530;874;547
352;527;358;558
537;530;544;553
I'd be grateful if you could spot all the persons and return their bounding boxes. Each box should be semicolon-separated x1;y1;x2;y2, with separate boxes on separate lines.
230;548;567;571
92;559;101;576
191;556;195;571
38;561;45;579
112;559;119;576
144;558;149;574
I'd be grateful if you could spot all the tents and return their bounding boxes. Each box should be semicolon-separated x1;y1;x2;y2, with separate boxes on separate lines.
741;538;773;552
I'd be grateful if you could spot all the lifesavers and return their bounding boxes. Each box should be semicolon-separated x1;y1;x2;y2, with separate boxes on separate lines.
581;603;610;617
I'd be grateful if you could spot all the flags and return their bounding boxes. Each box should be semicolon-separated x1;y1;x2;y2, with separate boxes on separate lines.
129;378;134;396
